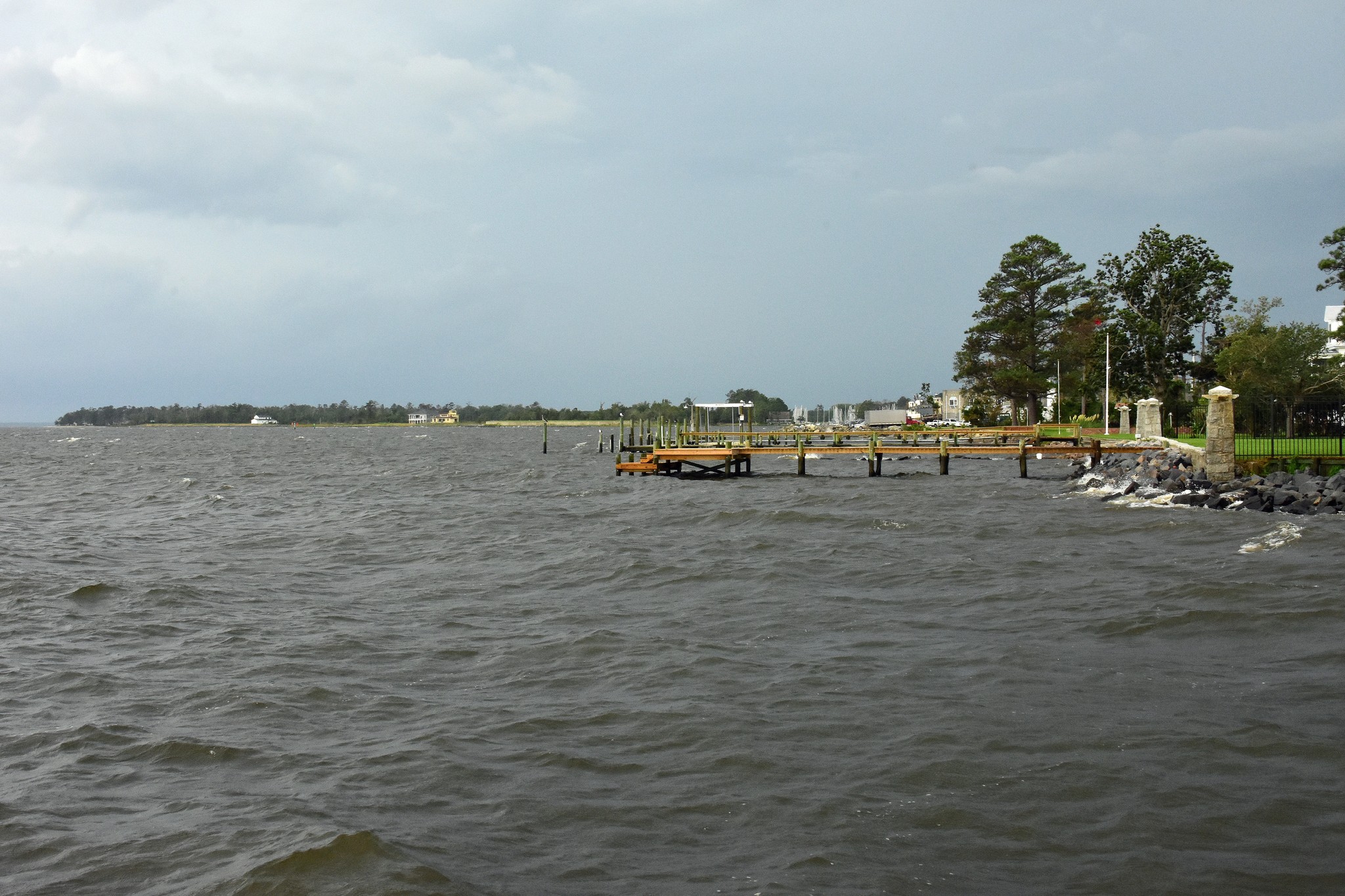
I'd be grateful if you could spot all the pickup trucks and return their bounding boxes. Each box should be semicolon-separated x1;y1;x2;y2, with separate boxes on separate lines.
927;420;942;427
939;418;960;427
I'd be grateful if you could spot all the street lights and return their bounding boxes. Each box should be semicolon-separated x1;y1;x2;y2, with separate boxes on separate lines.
684;404;695;440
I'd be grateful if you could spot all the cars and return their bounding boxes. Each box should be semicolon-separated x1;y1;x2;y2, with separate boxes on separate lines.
843;420;869;429
959;421;970;427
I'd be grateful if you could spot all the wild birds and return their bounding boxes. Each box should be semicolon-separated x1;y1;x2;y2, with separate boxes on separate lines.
620;413;624;417
599;429;602;433
1037;420;1041;424
541;414;548;423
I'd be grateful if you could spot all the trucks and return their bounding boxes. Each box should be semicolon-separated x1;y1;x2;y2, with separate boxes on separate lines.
864;409;918;428
